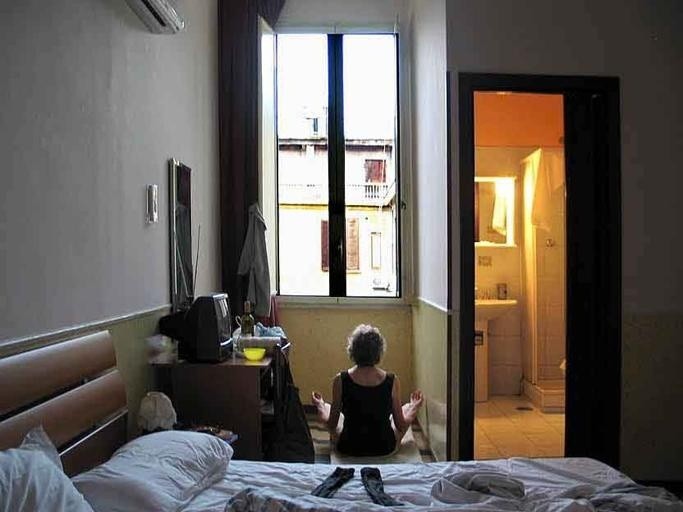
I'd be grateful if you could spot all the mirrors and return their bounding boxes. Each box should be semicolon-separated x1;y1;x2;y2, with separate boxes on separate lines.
474;176;517;247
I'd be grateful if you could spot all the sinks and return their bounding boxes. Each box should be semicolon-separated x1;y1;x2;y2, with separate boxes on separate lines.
475;299;518;319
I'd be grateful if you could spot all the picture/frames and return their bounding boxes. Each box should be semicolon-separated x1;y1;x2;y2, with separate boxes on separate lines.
168;158;194;314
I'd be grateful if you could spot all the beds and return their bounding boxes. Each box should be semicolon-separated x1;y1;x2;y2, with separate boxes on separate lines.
0;328;683;512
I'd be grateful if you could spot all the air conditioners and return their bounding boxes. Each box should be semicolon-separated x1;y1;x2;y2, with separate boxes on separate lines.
125;0;185;35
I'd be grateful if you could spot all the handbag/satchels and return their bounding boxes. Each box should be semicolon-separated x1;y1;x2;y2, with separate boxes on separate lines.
269;354;314;460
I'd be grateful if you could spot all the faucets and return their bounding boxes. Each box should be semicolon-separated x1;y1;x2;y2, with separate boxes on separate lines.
487;288;489;299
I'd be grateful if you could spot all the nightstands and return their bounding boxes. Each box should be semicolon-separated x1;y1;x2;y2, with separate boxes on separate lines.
175;424;240;458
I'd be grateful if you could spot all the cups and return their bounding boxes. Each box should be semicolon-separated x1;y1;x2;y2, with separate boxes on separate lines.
498;284;507;299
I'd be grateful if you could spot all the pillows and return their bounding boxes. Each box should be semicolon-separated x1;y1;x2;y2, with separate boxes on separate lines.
0;424;95;512
69;430;234;512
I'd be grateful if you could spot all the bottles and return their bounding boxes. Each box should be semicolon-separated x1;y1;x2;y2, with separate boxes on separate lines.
240;301;255;337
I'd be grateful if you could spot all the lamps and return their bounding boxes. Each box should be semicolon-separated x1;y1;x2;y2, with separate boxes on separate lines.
137;391;177;435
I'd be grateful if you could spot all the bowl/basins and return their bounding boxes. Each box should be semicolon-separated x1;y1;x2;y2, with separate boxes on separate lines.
243;348;265;361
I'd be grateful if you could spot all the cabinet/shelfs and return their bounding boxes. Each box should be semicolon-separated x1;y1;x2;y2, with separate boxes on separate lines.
147;340;293;460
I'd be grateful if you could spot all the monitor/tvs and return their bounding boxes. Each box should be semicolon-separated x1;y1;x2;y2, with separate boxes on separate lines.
159;292;234;363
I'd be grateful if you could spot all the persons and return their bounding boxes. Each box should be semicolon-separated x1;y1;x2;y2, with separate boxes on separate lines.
309;321;423;462
